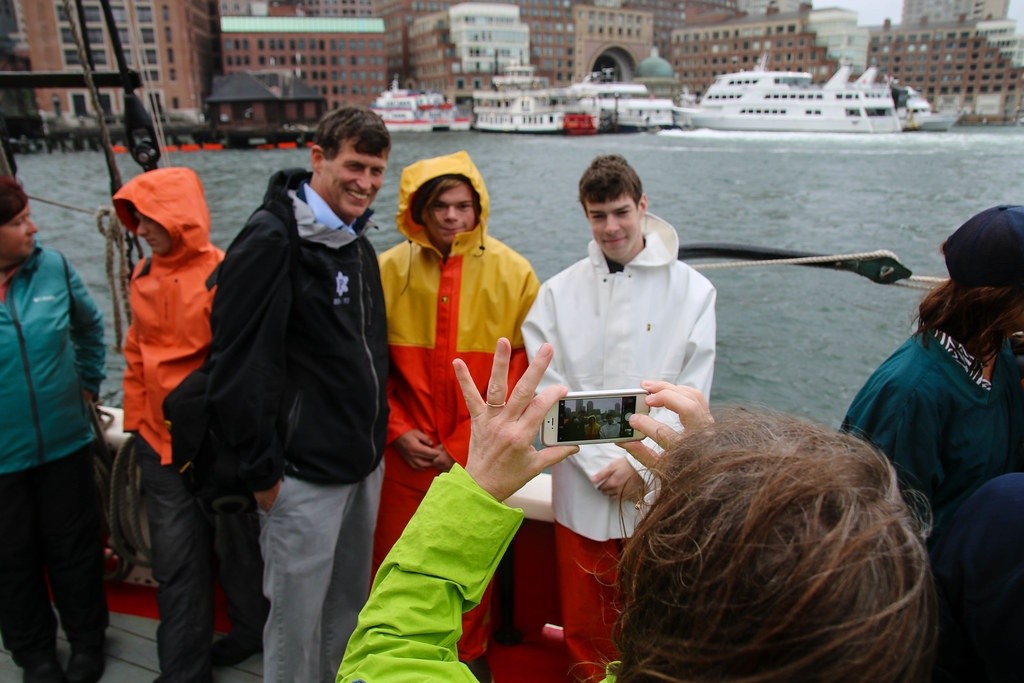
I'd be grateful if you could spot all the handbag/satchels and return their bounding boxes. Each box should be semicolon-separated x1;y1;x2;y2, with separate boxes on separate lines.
160;364;302;514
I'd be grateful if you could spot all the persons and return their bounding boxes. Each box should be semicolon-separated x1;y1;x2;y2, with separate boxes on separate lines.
113;163;270;683
518;155;716;683
368;150;548;683
839;202;1024;683
0;172;111;682
207;105;392;683
560;412;620;440
334;346;939;683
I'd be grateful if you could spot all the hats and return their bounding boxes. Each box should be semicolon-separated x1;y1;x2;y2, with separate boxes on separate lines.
944;204;1024;286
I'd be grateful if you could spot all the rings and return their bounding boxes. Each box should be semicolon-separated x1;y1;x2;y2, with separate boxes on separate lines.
486;400;506;407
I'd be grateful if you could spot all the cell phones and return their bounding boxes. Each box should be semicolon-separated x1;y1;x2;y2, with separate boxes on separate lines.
541;389;649;447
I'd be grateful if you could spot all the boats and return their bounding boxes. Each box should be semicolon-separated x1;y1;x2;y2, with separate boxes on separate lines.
371;73;479;134
469;47;968;134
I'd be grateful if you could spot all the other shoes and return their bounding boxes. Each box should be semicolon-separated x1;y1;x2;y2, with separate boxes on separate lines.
63;639;104;683
22;654;65;683
212;632;263;667
